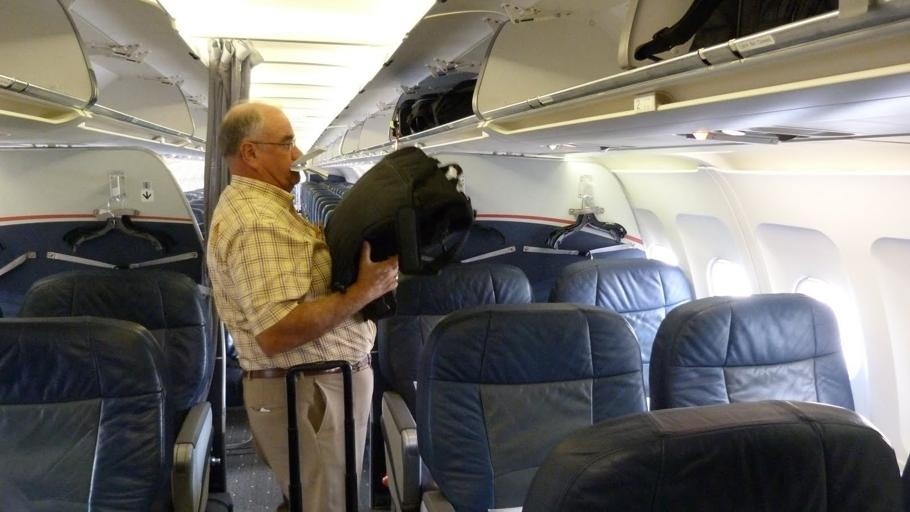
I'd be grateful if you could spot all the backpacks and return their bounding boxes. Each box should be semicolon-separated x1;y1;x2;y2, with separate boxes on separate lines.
324;147;476;321
634;0;837;68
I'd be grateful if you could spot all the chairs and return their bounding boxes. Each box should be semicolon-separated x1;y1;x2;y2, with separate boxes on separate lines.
0;315;171;512
296;172;908;512
16;270;219;508
178;185;209;235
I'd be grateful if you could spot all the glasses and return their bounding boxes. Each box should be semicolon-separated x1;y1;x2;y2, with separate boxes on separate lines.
250;140;294;151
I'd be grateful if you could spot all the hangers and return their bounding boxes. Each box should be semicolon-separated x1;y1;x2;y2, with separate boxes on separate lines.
68;196;164;258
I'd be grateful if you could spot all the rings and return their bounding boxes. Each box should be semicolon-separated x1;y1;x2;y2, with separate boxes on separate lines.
395;275;398;281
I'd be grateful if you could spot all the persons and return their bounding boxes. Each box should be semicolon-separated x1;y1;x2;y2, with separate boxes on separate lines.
203;102;403;511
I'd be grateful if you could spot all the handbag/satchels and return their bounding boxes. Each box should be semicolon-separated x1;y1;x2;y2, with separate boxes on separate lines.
390;68;477;139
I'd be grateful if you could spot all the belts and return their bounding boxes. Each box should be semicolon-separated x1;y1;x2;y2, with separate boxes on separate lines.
243;357;368;379
